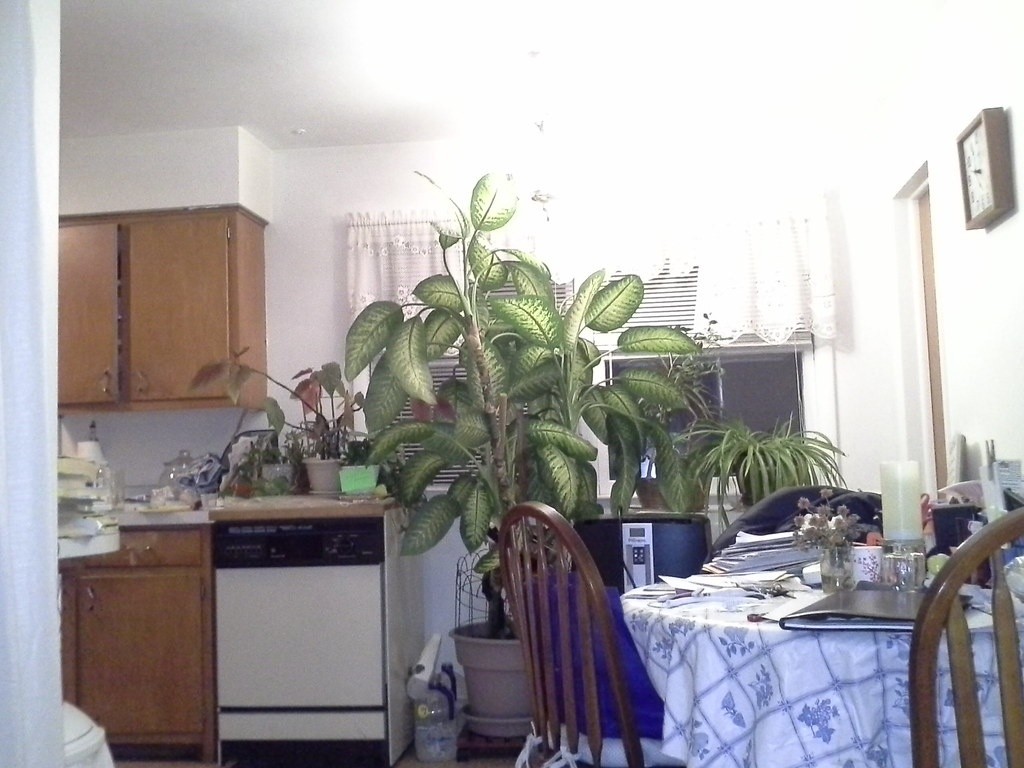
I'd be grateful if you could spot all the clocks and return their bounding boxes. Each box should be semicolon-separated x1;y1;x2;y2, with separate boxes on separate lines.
955;106;1015;232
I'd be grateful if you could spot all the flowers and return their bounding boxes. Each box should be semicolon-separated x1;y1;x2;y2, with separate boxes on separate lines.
789;488;861;570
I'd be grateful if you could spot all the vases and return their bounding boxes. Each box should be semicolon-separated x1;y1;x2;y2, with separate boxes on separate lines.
817;547;854;594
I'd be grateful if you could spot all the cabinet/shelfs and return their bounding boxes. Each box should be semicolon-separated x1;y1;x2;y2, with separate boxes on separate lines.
58;203;268;412
61;524;216;767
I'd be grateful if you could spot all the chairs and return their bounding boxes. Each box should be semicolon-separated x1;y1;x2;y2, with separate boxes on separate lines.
497;496;690;768
909;506;1024;768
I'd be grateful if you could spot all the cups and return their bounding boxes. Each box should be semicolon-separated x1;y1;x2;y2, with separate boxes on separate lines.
851;545;883;585
883;553;926;591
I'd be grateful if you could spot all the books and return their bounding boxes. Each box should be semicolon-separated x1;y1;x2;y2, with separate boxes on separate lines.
624;530;821;599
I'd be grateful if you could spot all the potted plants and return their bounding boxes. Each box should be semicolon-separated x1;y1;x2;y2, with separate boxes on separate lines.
192;343;402;499
342;165;705;736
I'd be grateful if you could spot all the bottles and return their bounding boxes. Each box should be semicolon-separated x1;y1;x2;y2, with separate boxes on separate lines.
413;662;466;763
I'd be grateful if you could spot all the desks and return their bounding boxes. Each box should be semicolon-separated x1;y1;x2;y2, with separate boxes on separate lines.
617;571;1024;767
